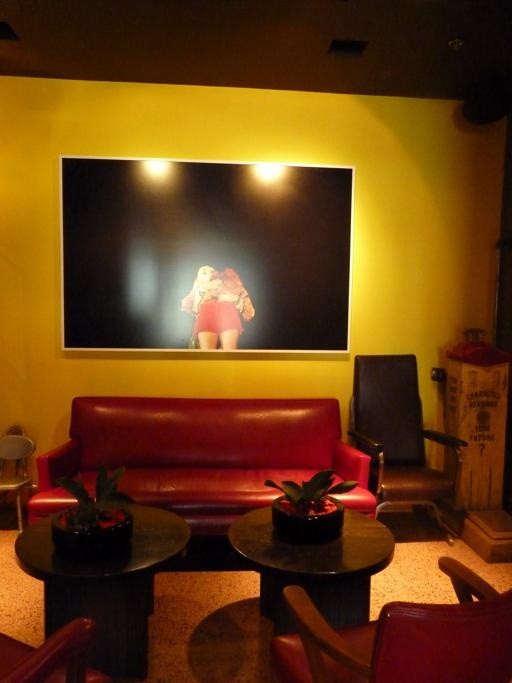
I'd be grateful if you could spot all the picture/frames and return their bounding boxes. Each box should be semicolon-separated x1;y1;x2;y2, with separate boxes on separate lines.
59;156;355;355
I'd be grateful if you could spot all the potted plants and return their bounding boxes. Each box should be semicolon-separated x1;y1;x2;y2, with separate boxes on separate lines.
50;466;133;557
263;470;358;546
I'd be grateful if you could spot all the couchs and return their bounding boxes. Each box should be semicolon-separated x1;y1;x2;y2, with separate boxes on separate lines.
26;396;377;542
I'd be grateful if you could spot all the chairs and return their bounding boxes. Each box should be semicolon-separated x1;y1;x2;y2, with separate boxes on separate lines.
346;354;468;547
270;557;512;682
0;617;114;683
0;424;36;533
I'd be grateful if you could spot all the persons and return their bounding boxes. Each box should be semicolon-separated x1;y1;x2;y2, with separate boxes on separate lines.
181;266;255;349
